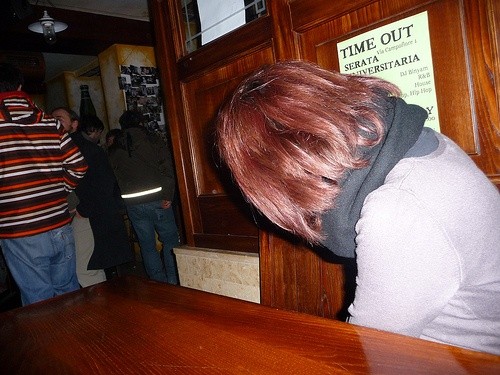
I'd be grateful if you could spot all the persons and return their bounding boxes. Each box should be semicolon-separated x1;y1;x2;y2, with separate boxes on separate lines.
211;59;500;357
0;62;88;308
28;101;137;287
107;109;179;285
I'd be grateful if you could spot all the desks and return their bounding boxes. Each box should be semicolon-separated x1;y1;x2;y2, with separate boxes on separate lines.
0;272;500;375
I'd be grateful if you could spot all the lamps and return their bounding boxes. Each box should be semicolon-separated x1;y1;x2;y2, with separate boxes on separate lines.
28;0;68;44
